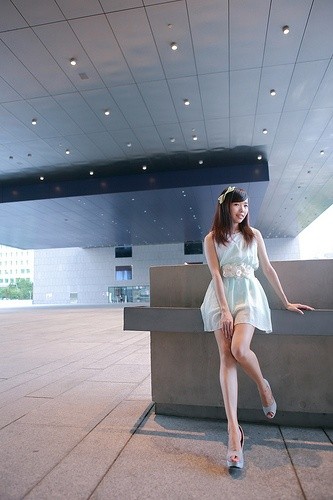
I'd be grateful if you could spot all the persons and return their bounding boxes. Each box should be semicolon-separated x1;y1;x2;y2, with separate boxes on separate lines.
199;186;316;470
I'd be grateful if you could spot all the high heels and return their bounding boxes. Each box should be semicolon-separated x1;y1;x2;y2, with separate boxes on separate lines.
226;425;244;474
262;379;277;419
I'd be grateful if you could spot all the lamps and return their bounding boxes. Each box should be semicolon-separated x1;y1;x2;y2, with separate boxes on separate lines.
184;99;190;106
270;90;276;96
282;26;290;35
70;58;77;66
32;119;37;126
104;109;111;116
170;42;178;51
